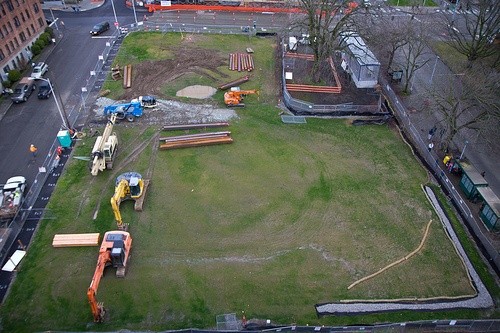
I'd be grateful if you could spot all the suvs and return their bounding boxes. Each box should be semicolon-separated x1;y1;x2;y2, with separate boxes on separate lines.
30;61;50;81
10;76;36;104
37;80;55;99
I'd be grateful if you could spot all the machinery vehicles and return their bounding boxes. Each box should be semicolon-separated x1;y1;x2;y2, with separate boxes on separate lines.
88;111;120;177
103;101;145;123
110;171;150;232
223;86;260;108
86;230;133;322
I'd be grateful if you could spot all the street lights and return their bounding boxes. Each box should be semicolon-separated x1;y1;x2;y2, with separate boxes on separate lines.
28;77;70;130
430;56;441;82
460;140;469;161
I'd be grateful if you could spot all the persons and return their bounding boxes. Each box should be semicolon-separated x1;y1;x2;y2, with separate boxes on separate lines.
428;128;434;140
428;142;434;152
30;144;38;157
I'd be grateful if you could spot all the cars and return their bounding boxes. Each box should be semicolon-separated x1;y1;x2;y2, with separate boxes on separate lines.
89;20;110;36
0;175;27;211
